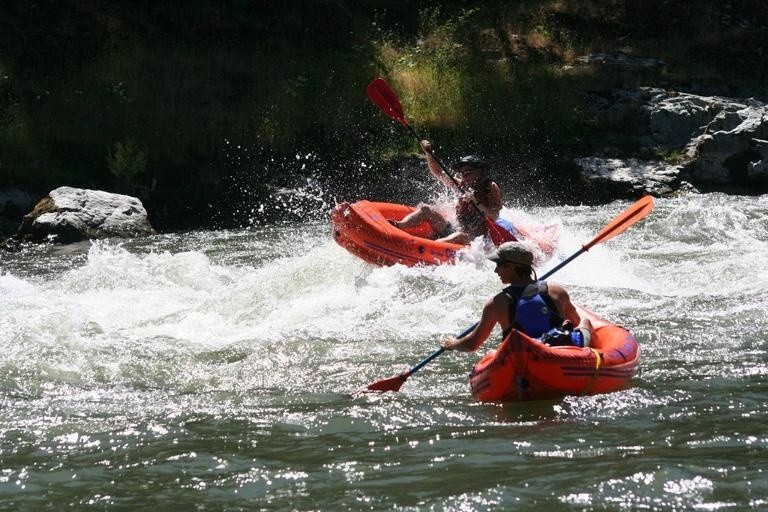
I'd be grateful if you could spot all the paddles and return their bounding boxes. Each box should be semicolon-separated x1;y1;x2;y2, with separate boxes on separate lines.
366;194;654;393
366;77;518;249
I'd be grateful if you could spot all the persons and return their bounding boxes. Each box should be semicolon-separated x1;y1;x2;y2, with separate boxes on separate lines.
389;139;504;242
442;240;594;354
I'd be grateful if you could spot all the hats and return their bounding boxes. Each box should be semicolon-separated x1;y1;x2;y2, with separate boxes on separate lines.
448;153;492;171
486;241;534;269
